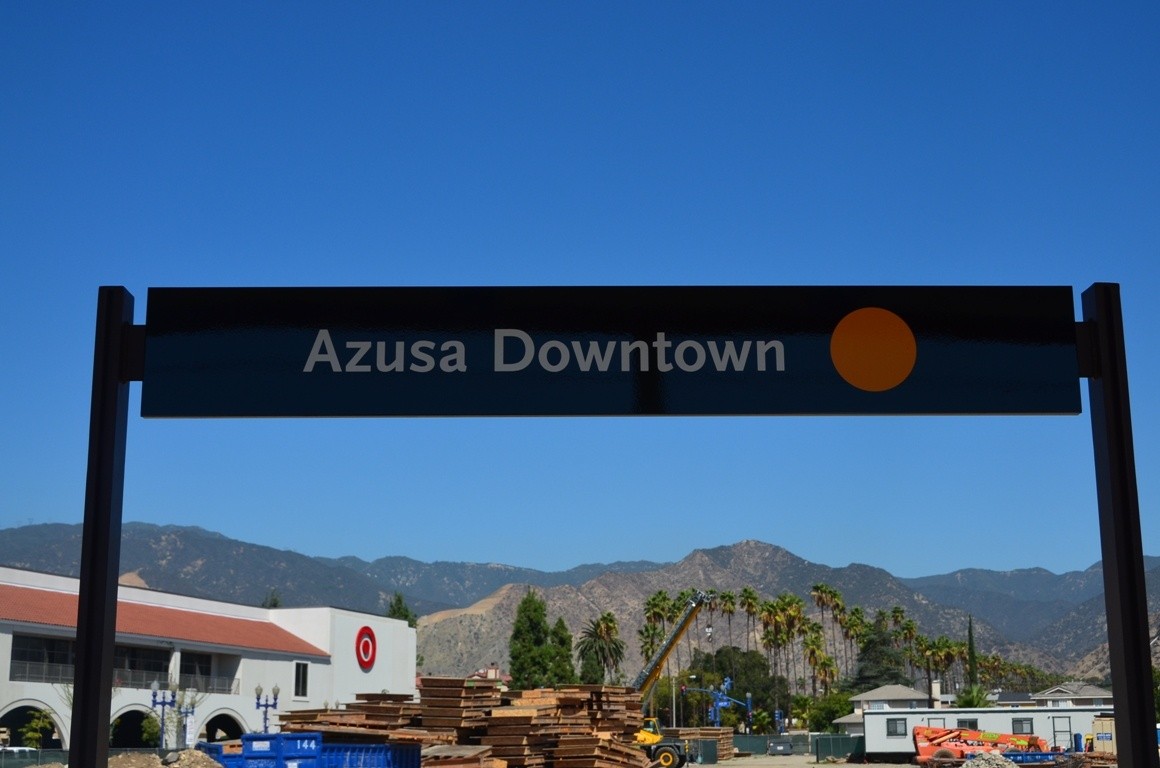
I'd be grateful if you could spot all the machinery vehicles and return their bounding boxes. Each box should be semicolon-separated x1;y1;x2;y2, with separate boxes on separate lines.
633;589;713;768
912;726;1083;768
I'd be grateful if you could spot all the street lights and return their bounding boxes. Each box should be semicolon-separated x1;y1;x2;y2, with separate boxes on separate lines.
175;695;196;749
254;682;279;734
150;679;178;748
709;684;725;727
323;700;340;710
746;691;753;734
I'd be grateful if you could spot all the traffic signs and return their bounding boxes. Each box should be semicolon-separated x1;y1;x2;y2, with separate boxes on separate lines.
140;285;1082;415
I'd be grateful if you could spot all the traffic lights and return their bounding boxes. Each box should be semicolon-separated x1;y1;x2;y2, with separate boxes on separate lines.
681;683;687;696
